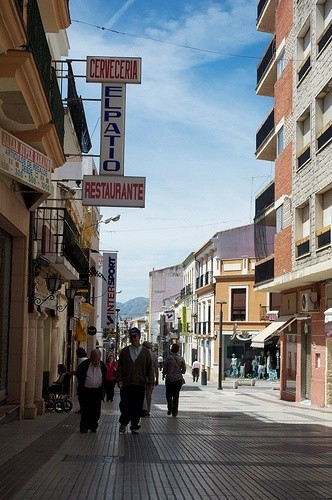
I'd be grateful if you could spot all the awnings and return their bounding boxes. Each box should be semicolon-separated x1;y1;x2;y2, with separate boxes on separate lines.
251;315;296;348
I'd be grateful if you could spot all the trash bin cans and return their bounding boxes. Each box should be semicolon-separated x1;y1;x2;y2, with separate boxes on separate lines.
201;369;208;385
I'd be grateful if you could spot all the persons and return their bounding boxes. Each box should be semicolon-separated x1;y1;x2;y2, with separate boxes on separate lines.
238;354;246;378
162;344;186;417
158;355;164;371
117;328;153;433
104;355;117;403
191;358;200;382
142;341;158;416
228;353;240;378
252;350;273;381
43;364;67;407
76;349;108;433
70;348;91;413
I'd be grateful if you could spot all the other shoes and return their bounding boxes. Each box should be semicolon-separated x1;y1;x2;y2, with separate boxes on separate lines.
168;409;172;415
172;409;177;417
80;428;88;433
132;429;139;434
90;428;96;433
142;410;149;417
118;424;126;433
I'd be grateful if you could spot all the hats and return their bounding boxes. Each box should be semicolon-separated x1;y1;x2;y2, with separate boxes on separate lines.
128;327;140;336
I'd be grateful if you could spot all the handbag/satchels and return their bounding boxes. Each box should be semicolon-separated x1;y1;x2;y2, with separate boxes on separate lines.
181;377;185;384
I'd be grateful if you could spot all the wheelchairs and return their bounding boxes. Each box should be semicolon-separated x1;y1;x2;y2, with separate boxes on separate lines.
45;372;73;413
